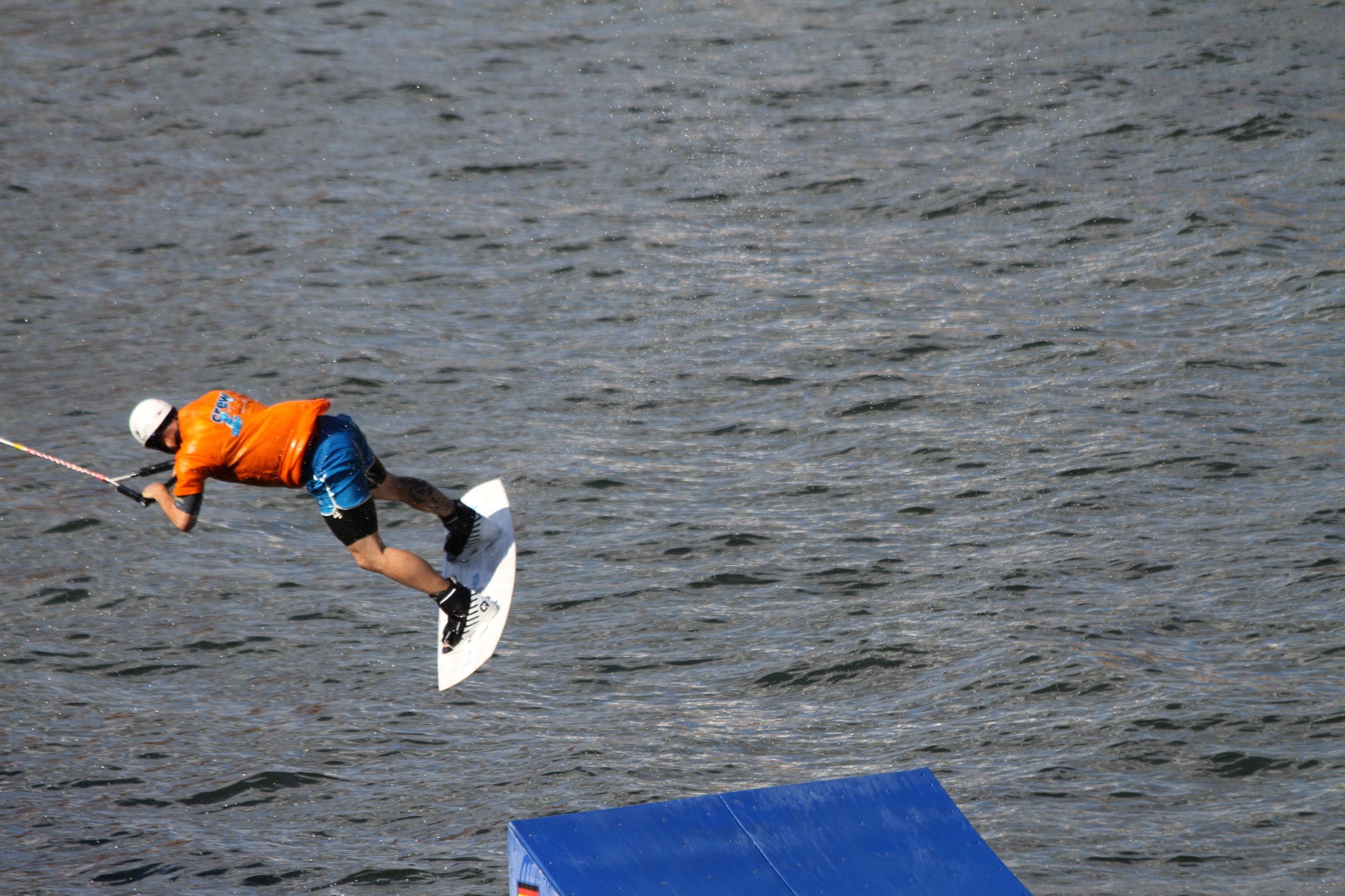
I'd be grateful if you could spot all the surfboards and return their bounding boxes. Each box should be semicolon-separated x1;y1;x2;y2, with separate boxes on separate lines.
433;477;521;703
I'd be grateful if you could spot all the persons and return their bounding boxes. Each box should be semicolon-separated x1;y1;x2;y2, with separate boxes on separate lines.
129;389;498;654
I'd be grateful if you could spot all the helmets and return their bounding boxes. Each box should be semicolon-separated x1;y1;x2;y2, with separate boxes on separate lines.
129;398;176;450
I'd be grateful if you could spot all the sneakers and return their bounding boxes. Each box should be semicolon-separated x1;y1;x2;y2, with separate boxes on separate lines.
429;574;498;656
440;499;503;564
